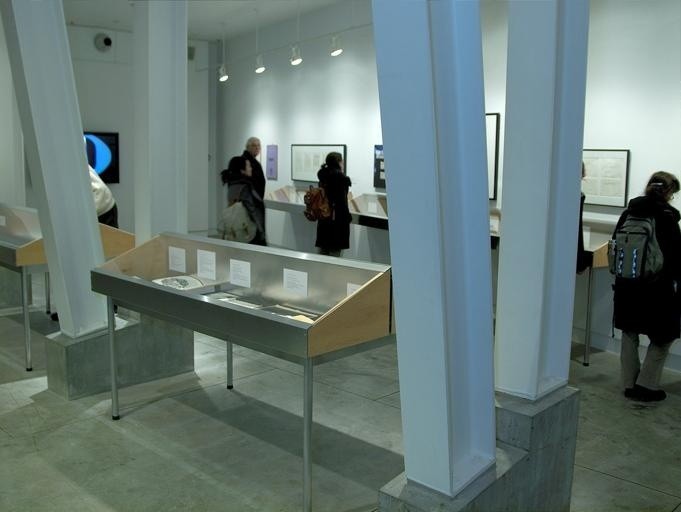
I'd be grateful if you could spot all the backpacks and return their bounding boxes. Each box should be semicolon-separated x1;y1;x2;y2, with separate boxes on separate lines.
303;184;331;222
606;212;665;280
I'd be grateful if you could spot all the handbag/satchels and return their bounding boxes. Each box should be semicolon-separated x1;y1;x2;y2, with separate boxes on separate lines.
216;202;257;243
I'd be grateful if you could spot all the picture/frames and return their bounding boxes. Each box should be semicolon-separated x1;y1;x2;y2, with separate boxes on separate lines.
485;113;501;201
291;144;347;184
583;149;630;208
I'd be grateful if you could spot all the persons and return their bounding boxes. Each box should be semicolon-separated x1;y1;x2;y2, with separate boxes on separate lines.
313;151;353;258
220;155;266;244
609;171;681;402
49;162;119;323
240;136;265;200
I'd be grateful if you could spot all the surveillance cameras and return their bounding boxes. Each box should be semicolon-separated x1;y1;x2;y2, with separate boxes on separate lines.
95;33;114;51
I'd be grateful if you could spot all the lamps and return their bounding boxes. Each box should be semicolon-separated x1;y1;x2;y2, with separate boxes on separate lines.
217;5;344;84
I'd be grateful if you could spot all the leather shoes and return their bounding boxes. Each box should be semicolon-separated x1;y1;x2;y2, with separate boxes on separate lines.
632;384;666;402
624;387;633;397
